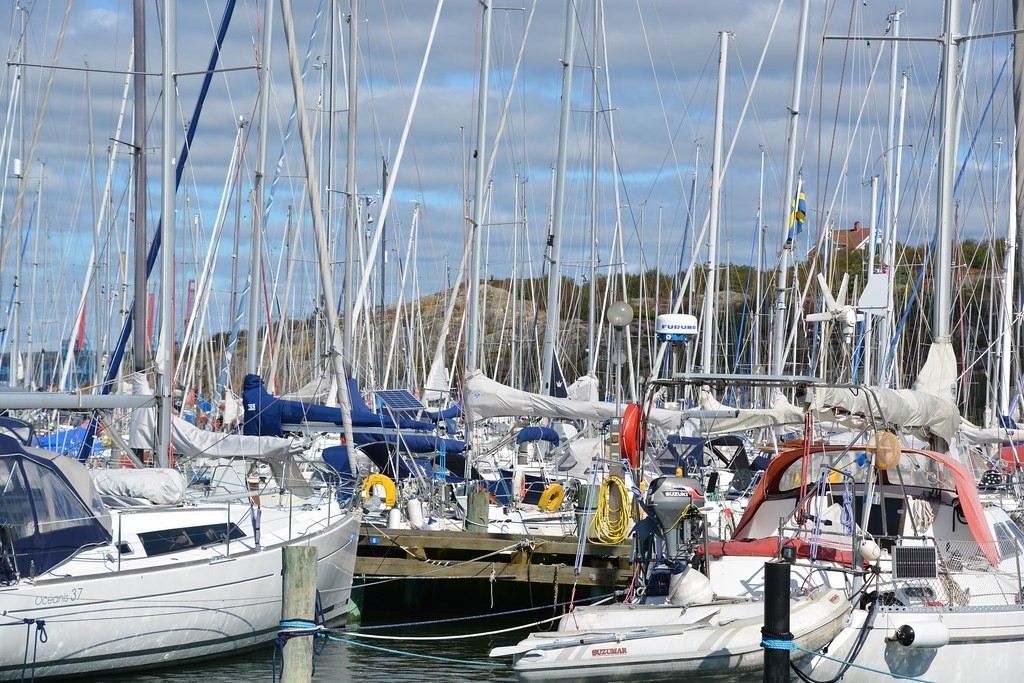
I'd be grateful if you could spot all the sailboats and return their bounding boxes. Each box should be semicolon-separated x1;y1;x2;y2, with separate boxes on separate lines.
3;0;1023;683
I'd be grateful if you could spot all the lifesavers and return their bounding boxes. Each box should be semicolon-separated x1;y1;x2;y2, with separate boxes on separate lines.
538;484;565;511
360;474;396;507
100;432;111;446
